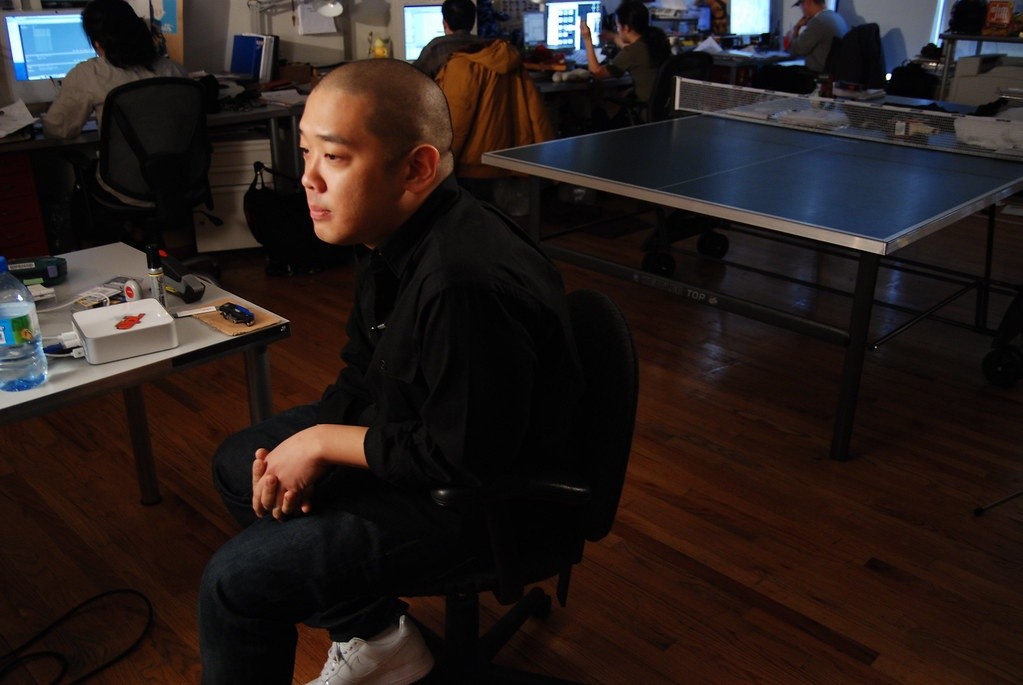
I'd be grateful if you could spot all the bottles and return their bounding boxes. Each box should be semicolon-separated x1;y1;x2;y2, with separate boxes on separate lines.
0;256;49;391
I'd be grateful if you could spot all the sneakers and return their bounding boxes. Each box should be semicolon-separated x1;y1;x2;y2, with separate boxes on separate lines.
301;614;434;685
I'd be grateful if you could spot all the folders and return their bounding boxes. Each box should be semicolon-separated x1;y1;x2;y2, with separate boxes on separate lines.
228;35;264;76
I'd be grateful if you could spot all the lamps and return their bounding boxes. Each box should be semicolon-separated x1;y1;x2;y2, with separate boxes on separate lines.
247;0;344;34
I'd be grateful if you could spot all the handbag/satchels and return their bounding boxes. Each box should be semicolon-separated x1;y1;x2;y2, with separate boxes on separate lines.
888;0;1022;114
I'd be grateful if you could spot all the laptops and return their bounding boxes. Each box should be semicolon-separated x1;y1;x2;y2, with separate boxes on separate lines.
695;4;736;36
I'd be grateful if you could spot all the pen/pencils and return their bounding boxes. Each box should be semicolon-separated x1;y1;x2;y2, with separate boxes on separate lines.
172;305;220;318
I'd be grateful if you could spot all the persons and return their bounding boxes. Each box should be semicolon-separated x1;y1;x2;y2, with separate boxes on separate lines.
38;0;191;254
580;0;673;128
412;0;496;81
197;57;577;685
751;0;849;93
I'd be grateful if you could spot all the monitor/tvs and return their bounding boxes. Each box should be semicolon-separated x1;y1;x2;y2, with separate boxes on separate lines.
544;0;603;49
0;8;99;103
726;0;771;35
522;11;545;49
390;0;478;65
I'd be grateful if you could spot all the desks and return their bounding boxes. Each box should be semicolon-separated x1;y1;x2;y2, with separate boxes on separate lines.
1;82;307;255
710;46;790;87
481;97;1023;464
0;241;291;516
533;55;637;138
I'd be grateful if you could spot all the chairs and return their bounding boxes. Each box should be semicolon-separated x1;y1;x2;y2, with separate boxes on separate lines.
424;35;555;178
43;74;221;264
615;51;713;127
780;22;886;89
385;289;639;685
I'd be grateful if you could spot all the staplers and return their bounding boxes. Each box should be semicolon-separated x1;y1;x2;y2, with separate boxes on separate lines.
149;247;206;305
